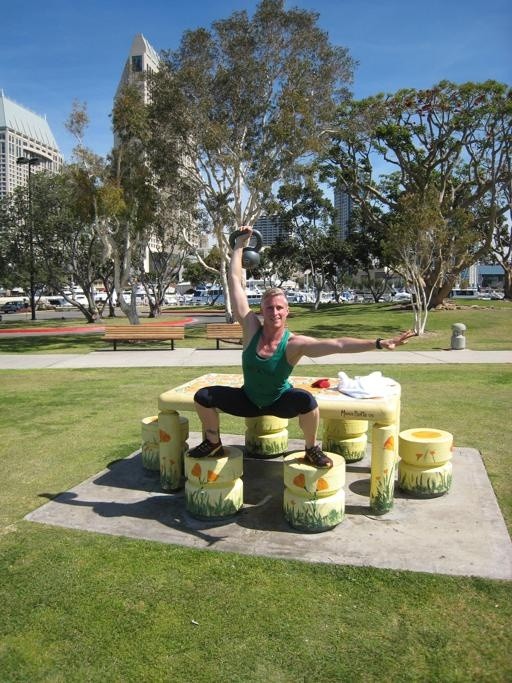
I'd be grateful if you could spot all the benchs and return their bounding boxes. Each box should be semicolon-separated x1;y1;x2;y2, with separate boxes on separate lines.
206;322;288;349
101;325;184;350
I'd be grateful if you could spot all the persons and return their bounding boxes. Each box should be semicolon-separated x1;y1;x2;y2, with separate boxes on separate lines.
184;225;419;469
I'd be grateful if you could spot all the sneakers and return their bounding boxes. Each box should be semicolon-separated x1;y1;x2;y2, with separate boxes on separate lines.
186;439;224;457
304;445;333;469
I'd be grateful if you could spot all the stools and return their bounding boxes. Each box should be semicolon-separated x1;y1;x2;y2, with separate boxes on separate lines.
244;414;289;459
184;446;243;521
141;416;189;473
398;428;453;499
321;418;368;462
283;450;346;532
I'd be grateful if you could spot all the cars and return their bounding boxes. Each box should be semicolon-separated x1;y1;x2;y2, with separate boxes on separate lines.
159;283;225;305
286;289;364;302
452;288;505;299
42;293;149;307
245;294;263;304
0;300;26;312
378;296;386;302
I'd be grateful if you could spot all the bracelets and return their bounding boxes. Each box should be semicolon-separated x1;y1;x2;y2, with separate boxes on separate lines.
375;337;385;350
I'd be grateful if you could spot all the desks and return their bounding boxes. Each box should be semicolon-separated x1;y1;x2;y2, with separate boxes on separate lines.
158;373;402;516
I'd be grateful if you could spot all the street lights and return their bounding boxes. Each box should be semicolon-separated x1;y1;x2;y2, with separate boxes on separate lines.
17;155;45;319
